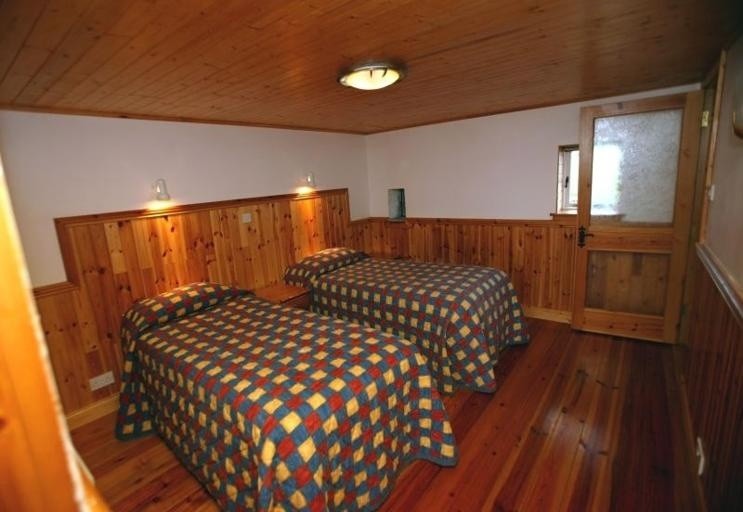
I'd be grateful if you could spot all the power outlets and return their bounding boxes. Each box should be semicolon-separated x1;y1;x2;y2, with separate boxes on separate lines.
90;371;115;391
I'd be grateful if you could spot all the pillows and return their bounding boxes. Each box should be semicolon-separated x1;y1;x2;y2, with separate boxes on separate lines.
287;247;368;277
124;281;240;327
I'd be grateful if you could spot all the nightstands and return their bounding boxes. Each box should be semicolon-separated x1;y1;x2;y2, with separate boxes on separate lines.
253;281;312;312
122;296;430;509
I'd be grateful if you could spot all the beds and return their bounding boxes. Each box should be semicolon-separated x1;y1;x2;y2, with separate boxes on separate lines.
283;255;518;384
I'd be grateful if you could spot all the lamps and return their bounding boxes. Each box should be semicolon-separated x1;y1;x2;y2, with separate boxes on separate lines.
335;61;406;92
151;178;171;201
